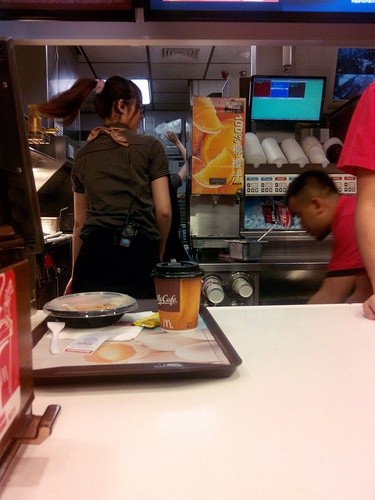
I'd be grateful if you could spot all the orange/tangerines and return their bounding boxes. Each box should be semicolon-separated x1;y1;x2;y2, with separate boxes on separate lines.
192;96;244;190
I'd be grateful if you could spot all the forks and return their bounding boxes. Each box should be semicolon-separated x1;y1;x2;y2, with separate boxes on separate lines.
47;321;65;355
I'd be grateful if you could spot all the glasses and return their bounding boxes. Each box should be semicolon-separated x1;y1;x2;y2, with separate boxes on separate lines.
126;102;145;114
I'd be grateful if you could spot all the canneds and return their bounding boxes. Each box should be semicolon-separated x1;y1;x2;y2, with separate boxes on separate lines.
262;203;292;229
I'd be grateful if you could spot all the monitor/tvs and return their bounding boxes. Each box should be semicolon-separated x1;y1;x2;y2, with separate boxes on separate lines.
249;75;327;123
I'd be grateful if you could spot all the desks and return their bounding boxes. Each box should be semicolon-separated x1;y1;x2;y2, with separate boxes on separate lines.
0;305;375;500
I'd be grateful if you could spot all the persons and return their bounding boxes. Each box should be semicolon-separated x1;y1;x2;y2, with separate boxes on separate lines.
36;75;173;300
164;130;190;263
285;167;372;304
336;81;375;321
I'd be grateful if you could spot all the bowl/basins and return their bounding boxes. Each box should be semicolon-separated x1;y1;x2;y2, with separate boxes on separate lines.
41;217;59;235
43;292;139;329
228;240;269;260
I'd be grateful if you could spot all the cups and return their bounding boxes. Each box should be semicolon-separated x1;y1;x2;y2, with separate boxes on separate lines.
278;204;292;228
203;278;224;303
261;203;278;224
151;261;204;330
232;278;253;298
